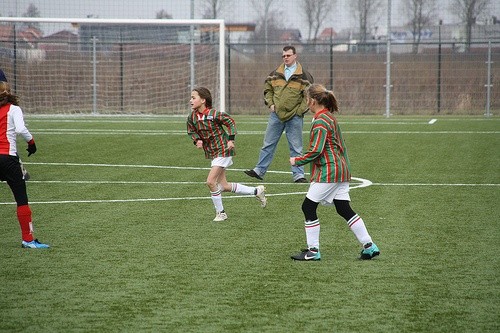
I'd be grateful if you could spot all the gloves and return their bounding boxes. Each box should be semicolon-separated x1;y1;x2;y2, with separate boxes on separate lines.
26;143;37;157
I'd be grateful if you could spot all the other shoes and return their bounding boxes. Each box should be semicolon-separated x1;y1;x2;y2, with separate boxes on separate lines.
295;178;307;182
244;170;263;180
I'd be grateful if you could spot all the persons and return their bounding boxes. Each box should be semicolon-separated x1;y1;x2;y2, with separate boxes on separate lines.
289;83;380;261
0;80;49;249
244;44;314;184
186;86;268;221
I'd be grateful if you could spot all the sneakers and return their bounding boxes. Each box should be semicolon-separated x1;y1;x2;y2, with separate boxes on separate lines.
360;242;380;260
255;185;267;208
21;239;49;249
212;212;227;222
291;248;321;260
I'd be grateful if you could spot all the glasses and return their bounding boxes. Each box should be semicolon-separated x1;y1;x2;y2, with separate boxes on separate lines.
282;54;294;58
